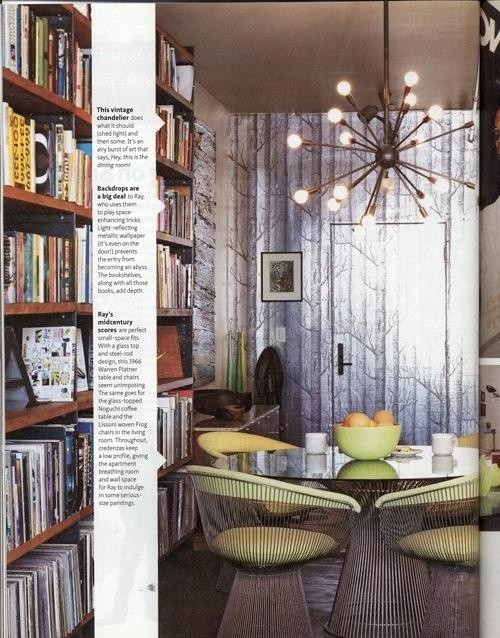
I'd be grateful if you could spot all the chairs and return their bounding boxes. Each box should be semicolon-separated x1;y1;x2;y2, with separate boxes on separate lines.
180;423;481;637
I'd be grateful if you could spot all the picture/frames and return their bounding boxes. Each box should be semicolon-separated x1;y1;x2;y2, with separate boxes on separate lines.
258;250;304;304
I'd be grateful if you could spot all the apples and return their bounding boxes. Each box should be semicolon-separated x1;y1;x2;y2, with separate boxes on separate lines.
342;410;395;427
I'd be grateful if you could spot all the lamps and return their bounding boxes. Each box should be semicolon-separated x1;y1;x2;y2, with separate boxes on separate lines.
281;0;488;235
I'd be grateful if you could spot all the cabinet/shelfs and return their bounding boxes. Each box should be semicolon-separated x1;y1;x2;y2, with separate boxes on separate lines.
149;17;200;560
1;1;96;637
187;401;281;536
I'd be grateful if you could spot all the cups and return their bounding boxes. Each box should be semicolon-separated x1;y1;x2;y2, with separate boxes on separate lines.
432;432;460;456
432;454;458;475
304;432;327;455
176;65;194;102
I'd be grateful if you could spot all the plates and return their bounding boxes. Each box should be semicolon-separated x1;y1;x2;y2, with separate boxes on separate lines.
391;449;424;459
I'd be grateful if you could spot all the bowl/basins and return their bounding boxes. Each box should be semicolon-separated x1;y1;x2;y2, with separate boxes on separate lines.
192;388;253;416
332;426;404;462
335;459;399;480
221;404;246;420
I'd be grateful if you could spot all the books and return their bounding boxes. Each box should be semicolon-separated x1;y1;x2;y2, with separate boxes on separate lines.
4;3;198;638
